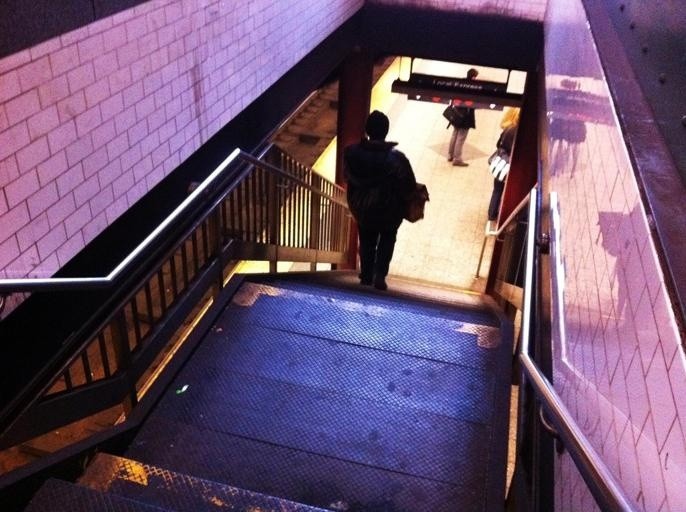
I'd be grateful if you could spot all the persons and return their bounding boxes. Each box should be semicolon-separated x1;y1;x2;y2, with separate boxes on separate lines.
484;106;521;236
548;79;586;180
342;110;416;290
447;68;478;166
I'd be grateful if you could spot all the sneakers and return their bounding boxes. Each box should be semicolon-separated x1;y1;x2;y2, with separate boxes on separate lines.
361;278;371;286
376;278;387;290
448;157;468;166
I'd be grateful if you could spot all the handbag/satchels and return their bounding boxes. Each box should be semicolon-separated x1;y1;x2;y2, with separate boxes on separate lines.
488;149;510;168
406;182;429;222
443;105;463;126
